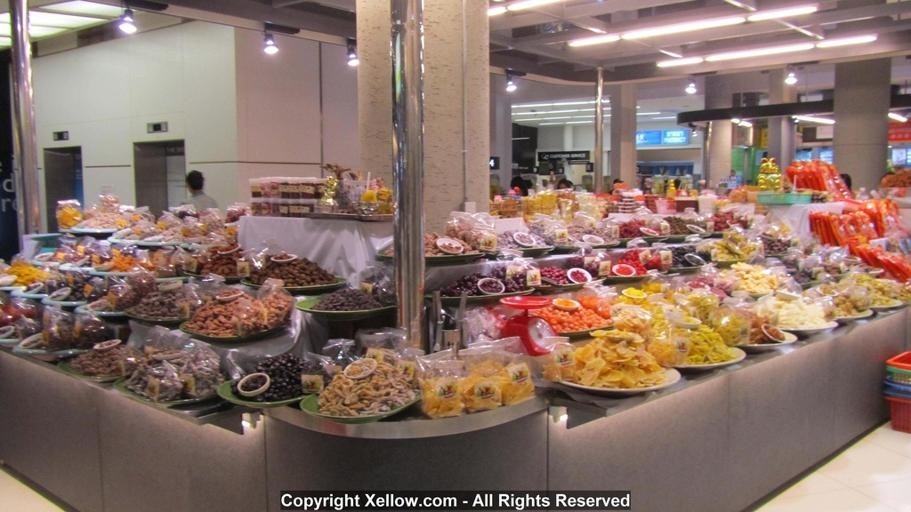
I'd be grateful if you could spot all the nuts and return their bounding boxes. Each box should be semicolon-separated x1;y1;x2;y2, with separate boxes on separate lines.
0;205;910;417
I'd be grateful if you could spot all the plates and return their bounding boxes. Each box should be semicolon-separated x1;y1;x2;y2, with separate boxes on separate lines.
111;371;217;407
611;262;636;277
293;293;398;324
436;236;464;255
512;231;536;248
566;266;593;285
537;273;889;398
298;387;422;426
215;374;307;411
685;223;706;235
475;276;506;295
683;252;705;268
0;244;349;386
582;233;605;246
639;226;659;239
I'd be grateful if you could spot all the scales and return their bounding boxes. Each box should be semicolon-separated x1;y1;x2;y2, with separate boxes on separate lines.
499;296;559;356
31;233;62;254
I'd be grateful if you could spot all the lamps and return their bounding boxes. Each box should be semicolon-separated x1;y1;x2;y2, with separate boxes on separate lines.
684;77;698;95
263;21;300;55
783;65;798;85
504;68;526;93
117;1;170;35
344;36;359;68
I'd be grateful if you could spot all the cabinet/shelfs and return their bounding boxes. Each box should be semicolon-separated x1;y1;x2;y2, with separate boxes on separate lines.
0;196;911;511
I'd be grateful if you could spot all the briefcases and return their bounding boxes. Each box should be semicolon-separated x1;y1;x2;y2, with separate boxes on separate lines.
882;351;911;435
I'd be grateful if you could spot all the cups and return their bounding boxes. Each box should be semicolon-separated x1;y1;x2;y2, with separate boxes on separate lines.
654;196;669;215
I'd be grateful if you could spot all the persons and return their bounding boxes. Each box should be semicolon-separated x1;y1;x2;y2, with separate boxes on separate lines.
558;178;572;188
180;170;219;209
